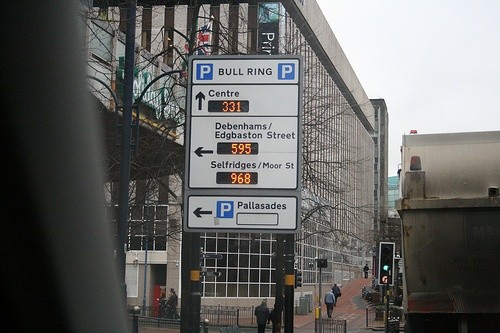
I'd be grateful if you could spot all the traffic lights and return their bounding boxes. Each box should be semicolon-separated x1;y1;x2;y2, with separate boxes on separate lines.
294;269;303;288
378;241;395;286
318;258;327;268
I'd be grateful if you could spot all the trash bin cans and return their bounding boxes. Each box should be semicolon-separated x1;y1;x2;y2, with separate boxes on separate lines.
299;294;313;314
386;318;400;333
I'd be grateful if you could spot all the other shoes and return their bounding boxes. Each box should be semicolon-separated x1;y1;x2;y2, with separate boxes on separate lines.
329;315;332;318
334;304;336;306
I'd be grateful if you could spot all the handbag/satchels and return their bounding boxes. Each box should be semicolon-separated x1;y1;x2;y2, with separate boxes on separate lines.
339;293;341;297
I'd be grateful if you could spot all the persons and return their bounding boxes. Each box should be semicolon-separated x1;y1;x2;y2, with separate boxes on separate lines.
363;264;369;278
332;284;340;306
254;300;276;333
167;288;180;319
324;290;336;318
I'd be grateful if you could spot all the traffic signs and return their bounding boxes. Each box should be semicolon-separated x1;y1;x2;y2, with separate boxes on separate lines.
183;52;303;235
